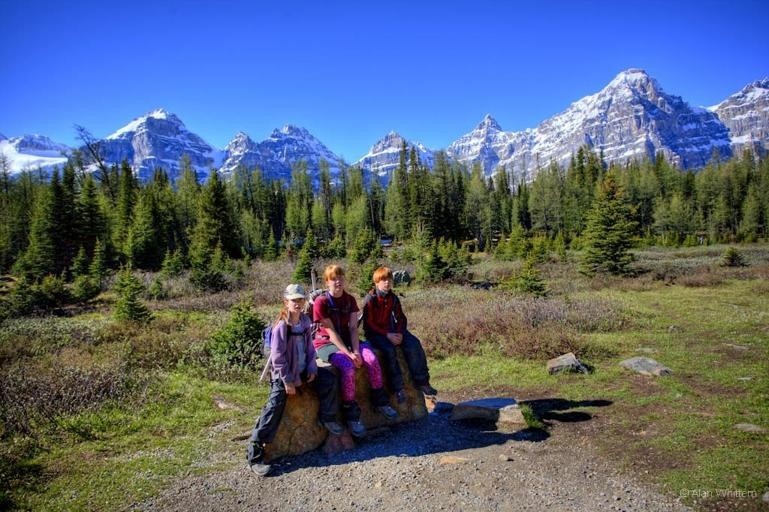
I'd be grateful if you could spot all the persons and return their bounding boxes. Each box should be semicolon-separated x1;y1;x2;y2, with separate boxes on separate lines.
311;264;399;438
245;284;343;475
363;266;437;405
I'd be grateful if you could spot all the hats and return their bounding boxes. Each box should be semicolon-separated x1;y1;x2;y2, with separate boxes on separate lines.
284;284;305;300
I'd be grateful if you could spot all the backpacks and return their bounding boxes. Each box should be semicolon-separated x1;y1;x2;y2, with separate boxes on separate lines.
304;288;340;340
262;319;292;356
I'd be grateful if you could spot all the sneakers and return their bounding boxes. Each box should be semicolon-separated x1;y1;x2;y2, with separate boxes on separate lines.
396;388;407;404
317;418;343;435
377;406;397;419
348;418;365;438
252;461;274;477
415;383;437;395
